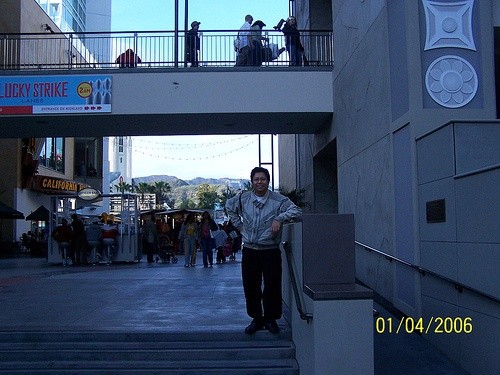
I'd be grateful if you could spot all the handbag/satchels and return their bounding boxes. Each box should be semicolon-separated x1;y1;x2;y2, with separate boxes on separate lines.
234;38;241;52
303;55;309;67
229;230;238;239
261;48;272;60
210;225;221;238
240;45;250;56
269;43;279;59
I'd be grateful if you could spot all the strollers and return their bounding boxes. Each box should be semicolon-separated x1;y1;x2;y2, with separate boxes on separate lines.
155;233;178;264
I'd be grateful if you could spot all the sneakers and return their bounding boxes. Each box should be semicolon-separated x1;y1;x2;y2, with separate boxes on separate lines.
265;319;280;334
244;319;264;335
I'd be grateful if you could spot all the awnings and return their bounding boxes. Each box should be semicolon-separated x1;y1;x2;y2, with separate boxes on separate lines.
28;165;92;195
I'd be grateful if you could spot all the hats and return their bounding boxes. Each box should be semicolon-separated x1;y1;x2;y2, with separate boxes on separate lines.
191;21;200;27
92;217;101;222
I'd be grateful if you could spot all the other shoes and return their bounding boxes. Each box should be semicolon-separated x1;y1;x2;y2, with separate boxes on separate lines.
216;262;221;264
97;253;101;258
210;262;213;266
148;260;155;263
191;264;195;267
204;265;207;268
222;261;225;263
185;264;189;267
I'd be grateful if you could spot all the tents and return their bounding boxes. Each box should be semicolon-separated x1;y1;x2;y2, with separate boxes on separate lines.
0;202;25;219
24;205;60;220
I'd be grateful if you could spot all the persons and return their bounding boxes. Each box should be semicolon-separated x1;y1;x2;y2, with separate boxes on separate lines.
143;216;157;263
68;214;86;267
275;15;305;67
225;166;302;334
83;215;237;259
22;227;48;258
214;224;230;264
197;211;218;267
179;213;198;267
115;49;141;68
237;15;253;65
52;219;72;260
186;21;201;67
101;220;119;258
251;20;269;65
86;219;103;258
